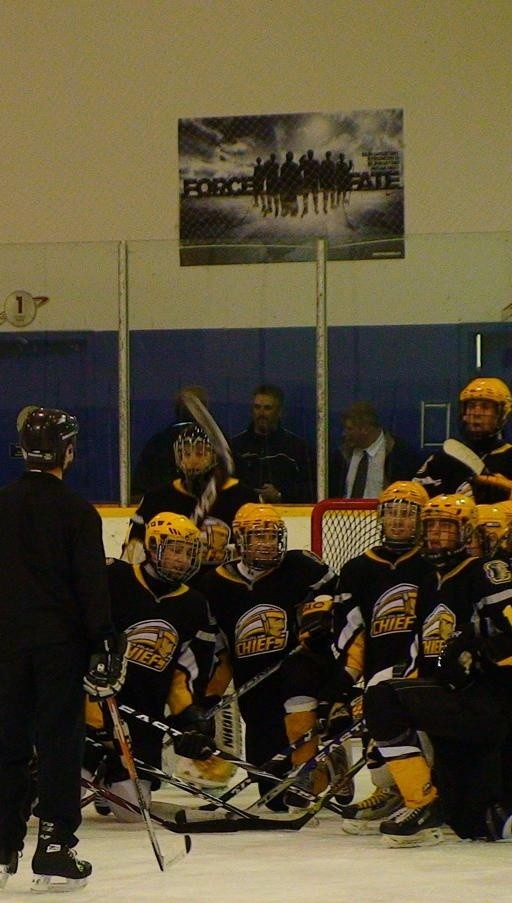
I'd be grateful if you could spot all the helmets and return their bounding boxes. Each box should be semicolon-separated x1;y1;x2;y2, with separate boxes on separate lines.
459;376;512;440
421;493;477;562
377;480;428;548
477;504;507;557
171;421;215;477
233;503;288;569
499;499;512;548
145;511;203;584
17;404;77;471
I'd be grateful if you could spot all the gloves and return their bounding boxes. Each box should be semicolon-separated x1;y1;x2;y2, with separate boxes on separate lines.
298;595;331;649
170;694;216;739
439;632;498;683
322;672;354;736
170;702;215;759
84;637;133;701
103;722;135;765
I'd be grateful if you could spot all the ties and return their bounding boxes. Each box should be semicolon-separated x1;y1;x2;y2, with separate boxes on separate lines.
350;451;370;497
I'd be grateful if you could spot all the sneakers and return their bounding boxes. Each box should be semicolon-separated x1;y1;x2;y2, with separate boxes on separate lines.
0;805;30;852
340;786;405;819
325;747;356;803
280;765;317;807
33;822;92;879
93;788;111;814
380;799;442;833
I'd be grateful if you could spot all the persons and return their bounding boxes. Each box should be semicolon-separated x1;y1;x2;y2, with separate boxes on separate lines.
464;505;512;671
329;401;430;499
231;382;312;504
299;482;428;834
412;380;512;504
202;504;355;813
364;493;477;848
0;405;106;893
135;385;227;493
119;422;262;563
88;513;207;822
253;149;352;220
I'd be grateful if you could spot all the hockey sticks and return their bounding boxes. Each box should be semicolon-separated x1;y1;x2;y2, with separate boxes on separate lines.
81;778;235;834
235;758;367;831
150;727;315;825
443;437;493;479
107;695;192;871
132;757;320;829
179;388;233;528
174;717;366;823
